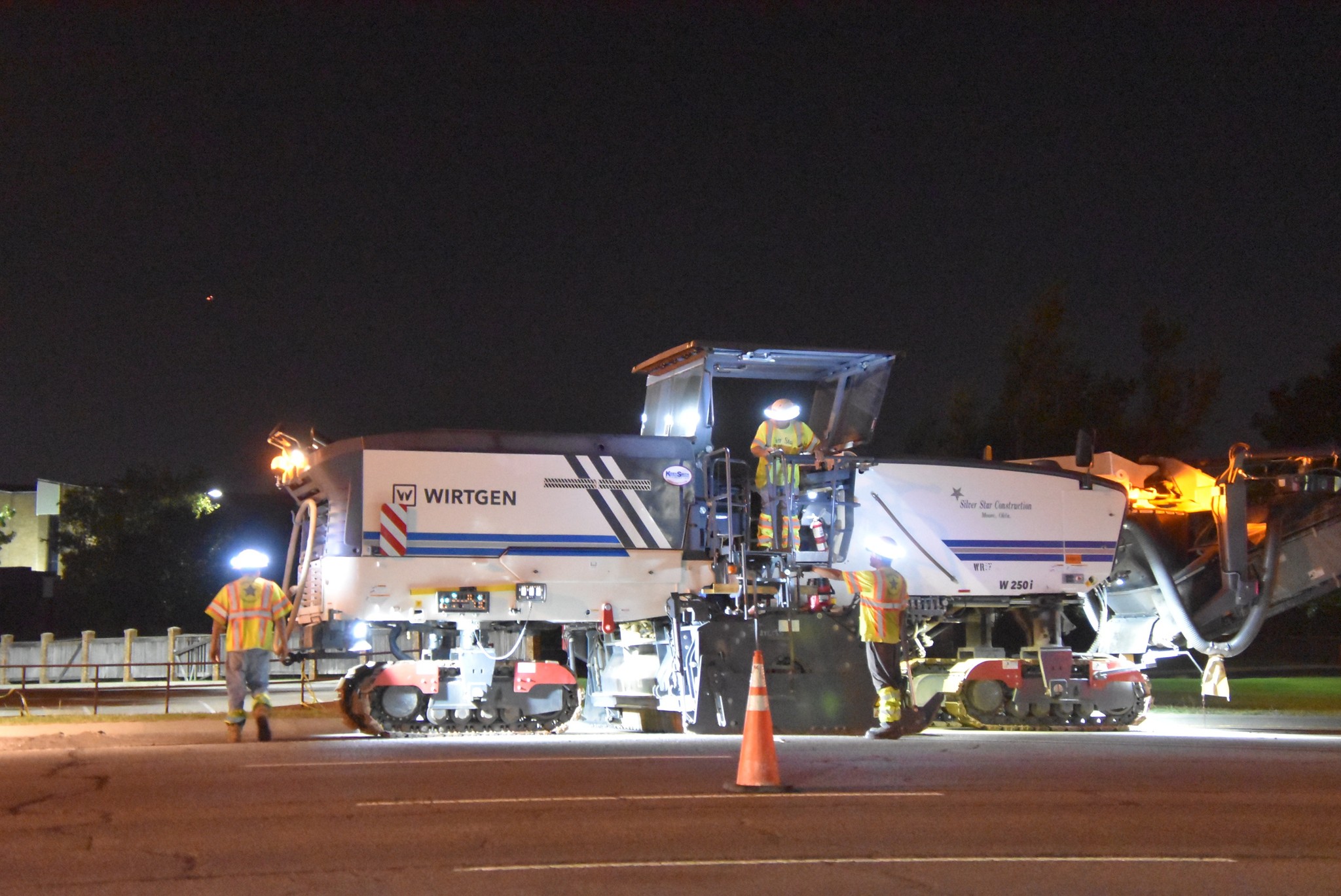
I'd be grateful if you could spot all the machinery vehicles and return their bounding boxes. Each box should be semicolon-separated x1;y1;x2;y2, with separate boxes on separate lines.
262;338;1341;738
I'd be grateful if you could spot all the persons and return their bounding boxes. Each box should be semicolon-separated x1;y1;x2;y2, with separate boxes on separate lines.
751;398;826;558
204;549;295;742
795;536;907;740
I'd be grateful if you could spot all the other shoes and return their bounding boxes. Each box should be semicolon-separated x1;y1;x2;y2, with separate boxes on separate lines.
864;719;905;739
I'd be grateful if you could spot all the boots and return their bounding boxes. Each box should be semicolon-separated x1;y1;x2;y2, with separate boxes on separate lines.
225;707;247;745
252;701;273;742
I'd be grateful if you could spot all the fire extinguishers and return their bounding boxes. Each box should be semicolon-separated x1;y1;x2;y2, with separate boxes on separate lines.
807;514;829;552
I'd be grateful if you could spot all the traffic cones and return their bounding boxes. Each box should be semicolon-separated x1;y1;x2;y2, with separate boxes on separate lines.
723;647;797;792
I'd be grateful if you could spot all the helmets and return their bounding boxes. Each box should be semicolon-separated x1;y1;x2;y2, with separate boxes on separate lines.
764;399;800;421
866;537;903;560
229;549;269;571
